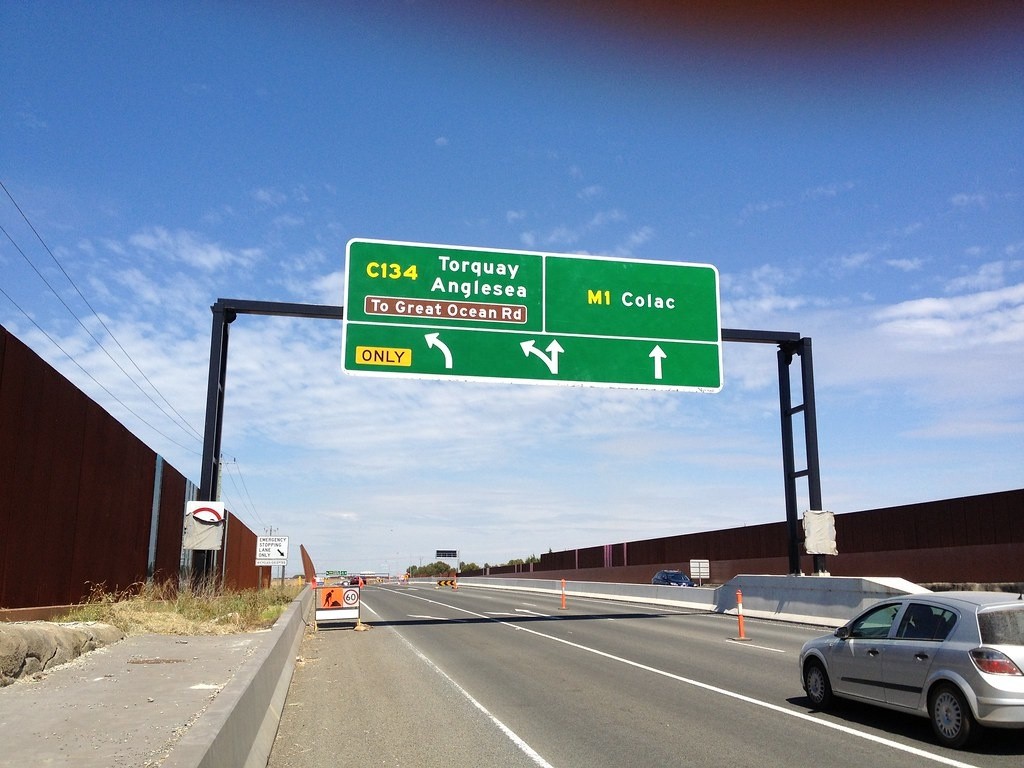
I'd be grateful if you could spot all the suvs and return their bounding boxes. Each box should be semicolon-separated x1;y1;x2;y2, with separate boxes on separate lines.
651;570;698;587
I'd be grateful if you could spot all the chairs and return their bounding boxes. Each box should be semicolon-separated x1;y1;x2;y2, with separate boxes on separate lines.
920;615;948;639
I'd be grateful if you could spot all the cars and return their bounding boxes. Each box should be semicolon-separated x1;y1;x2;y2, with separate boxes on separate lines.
351;576;367;585
798;591;1024;749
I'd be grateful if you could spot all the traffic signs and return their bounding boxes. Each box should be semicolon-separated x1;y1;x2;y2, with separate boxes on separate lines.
255;536;289;560
341;238;723;395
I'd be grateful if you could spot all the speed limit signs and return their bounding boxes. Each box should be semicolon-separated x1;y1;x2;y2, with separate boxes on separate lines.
343;590;359;606
